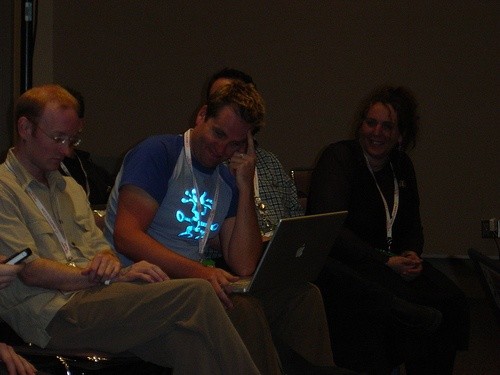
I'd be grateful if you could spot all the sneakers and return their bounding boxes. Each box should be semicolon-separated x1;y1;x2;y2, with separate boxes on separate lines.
381;293;443;337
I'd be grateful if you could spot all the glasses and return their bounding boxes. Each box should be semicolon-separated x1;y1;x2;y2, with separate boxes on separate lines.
34;124;81;148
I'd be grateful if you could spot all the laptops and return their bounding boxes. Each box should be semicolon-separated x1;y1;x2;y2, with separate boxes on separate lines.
226;211;349;294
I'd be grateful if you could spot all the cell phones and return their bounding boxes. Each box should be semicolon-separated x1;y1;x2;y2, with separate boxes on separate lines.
5;249;32;265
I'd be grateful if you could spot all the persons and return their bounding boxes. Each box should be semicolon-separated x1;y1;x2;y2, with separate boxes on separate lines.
57;83;112;205
104;82;337;375
202;68;307;276
306;85;470;375
0;83;284;375
